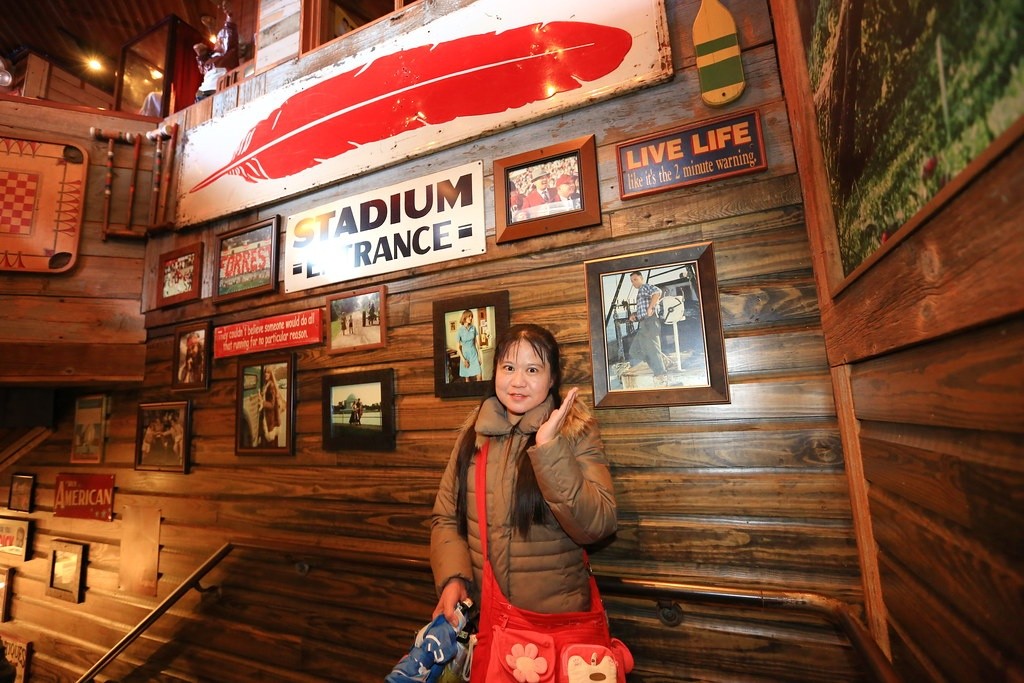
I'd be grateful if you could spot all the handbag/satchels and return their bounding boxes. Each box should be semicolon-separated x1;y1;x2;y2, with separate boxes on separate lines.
464;560;635;683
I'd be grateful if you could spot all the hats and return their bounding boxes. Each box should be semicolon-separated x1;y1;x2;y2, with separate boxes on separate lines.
531;170;551;181
555;174;576;186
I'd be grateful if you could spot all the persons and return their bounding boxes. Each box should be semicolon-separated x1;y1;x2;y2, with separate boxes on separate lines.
193;0;239;103
507;155;581;224
348;315;353;334
431;323;616;683
258;364;280;448
142;411;183;465
629;271;667;376
14;527;25;548
369;303;374;325
456;309;482;382
183;332;204;384
349;398;363;426
341;311;346;335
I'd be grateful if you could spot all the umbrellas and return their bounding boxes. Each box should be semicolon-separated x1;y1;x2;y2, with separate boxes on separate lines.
434;615;472;683
385;597;476;683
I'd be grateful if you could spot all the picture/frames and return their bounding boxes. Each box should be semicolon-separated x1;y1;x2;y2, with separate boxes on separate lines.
133;400;190;473
46;540;87;605
0;516;33;562
7;474;36;514
69;394;107;464
171;320;213;392
583;241;731;410
212;214;281;303
493;134;602;246
0;564;14;623
432;290;510;399
321;367;394;450
155;241;205;307
234;351;297;455
326;286;387;356
